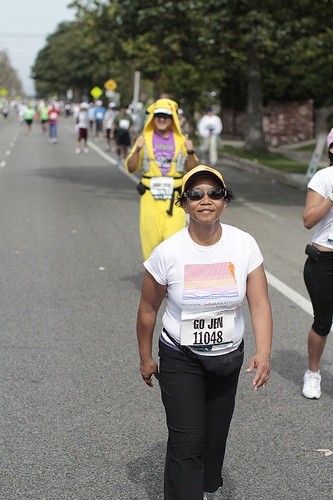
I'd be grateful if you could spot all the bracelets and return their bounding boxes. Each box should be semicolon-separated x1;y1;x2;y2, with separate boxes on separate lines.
187;149;195;154
326;193;333;202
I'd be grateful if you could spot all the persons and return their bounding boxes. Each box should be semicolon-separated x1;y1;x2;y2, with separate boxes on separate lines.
124;98;199;261
0;93;222;165
137;165;273;500
302;128;333;399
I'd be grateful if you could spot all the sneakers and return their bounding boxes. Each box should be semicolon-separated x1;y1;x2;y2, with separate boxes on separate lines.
302;368;321;399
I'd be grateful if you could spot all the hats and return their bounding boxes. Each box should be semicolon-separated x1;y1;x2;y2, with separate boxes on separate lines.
153;109;172;118
181;165;226;196
327;128;333;148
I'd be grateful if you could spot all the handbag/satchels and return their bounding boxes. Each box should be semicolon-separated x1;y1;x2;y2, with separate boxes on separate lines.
163;328;243;377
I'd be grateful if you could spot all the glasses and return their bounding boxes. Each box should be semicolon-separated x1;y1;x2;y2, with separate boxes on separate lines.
186;188;226;200
